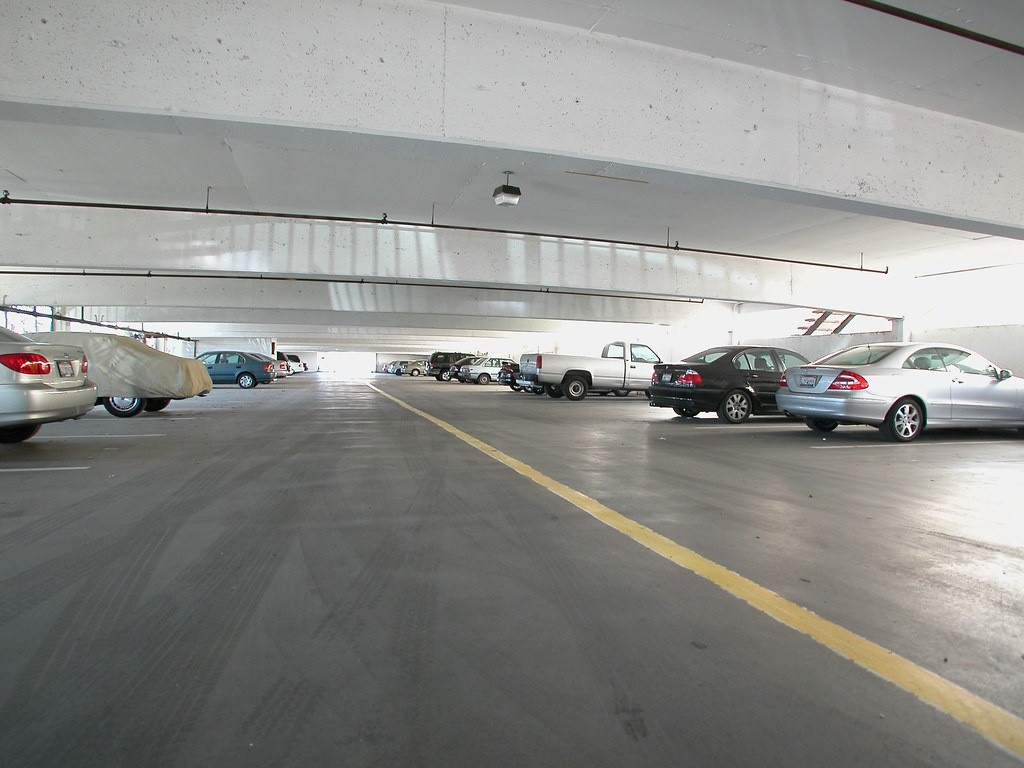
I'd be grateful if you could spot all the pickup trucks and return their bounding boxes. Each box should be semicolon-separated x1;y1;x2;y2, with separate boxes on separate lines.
515;339;665;399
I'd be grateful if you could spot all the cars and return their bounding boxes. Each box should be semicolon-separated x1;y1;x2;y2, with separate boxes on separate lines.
775;341;1023;445
22;331;213;419
0;326;98;445
192;350;278;389
647;345;810;424
286;358;305;373
250;352;288;378
382;352;543;394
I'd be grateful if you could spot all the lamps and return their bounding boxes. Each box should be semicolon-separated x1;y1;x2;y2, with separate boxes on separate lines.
492;171;521;208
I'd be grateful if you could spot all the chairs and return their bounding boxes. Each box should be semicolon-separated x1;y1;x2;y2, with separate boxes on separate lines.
754;357;772;371
913;357;932;370
220;358;229;363
634;358;647;361
